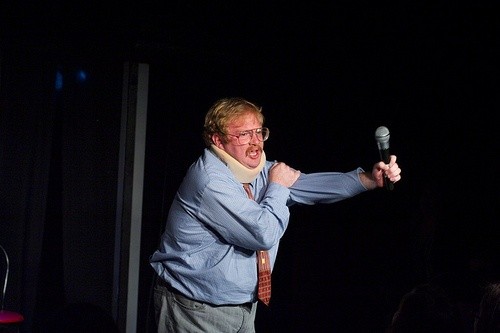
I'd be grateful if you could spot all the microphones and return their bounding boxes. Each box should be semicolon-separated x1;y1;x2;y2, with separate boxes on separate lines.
375;126;394;191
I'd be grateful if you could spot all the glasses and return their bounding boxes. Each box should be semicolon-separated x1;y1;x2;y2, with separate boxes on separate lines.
222;127;269;145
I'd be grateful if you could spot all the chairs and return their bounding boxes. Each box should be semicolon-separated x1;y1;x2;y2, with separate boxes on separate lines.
0;246;24;333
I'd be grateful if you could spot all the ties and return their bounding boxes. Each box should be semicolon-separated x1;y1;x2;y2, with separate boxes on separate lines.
241;182;271;307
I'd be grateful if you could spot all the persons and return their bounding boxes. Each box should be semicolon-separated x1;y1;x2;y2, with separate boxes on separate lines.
33;279;500;333
151;97;400;333
